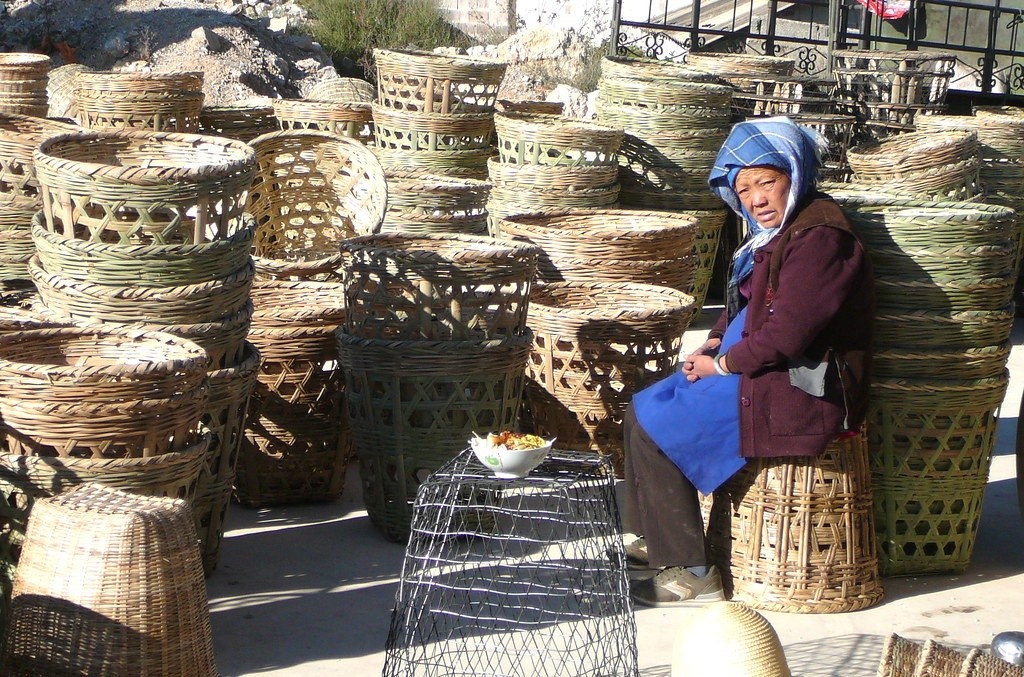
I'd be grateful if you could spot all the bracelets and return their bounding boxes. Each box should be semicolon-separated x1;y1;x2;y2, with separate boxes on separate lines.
713;352;733;376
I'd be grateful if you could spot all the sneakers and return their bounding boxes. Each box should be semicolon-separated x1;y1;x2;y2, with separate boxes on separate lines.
604;534;663;568
623;563;726;605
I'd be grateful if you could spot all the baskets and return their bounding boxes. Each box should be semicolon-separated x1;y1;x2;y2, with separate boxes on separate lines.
687;50;831;124
849;124;985;204
717;424;883;616
75;69;204;135
594;54;733;325
0;324;215;674
224;128;388;503
832;202;1015;582
832;47;955;138
517;279;696;465
1;52;77;306
382;446;638;676
915;105;1022;277
371;45;506;234
274;96;373;142
747;112;855;178
204;104;275;144
485;109;621;234
26;128;259;573
337;228;542;542
500;209;697;288
873;632;1022;676
523;279;696;475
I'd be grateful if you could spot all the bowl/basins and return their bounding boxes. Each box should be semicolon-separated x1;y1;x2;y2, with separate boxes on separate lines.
469;439;552;477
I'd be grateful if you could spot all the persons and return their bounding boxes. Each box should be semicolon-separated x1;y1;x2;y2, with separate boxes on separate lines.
607;111;875;606
